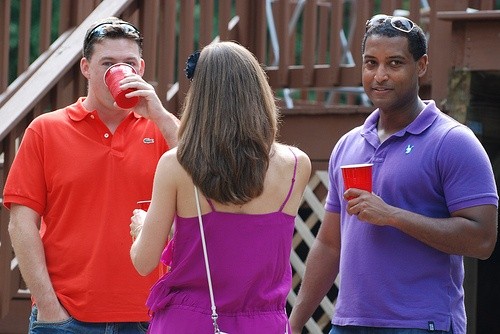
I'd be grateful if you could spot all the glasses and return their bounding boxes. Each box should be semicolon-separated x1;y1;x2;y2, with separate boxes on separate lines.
365;14;417;32
87;22;141;37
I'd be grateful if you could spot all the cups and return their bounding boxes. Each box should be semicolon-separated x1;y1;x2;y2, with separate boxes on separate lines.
340;163;374;198
103;62;141;109
137;199;153;212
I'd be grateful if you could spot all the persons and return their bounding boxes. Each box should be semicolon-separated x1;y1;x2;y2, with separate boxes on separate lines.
2;16;182;334
130;41;311;334
290;14;500;334
